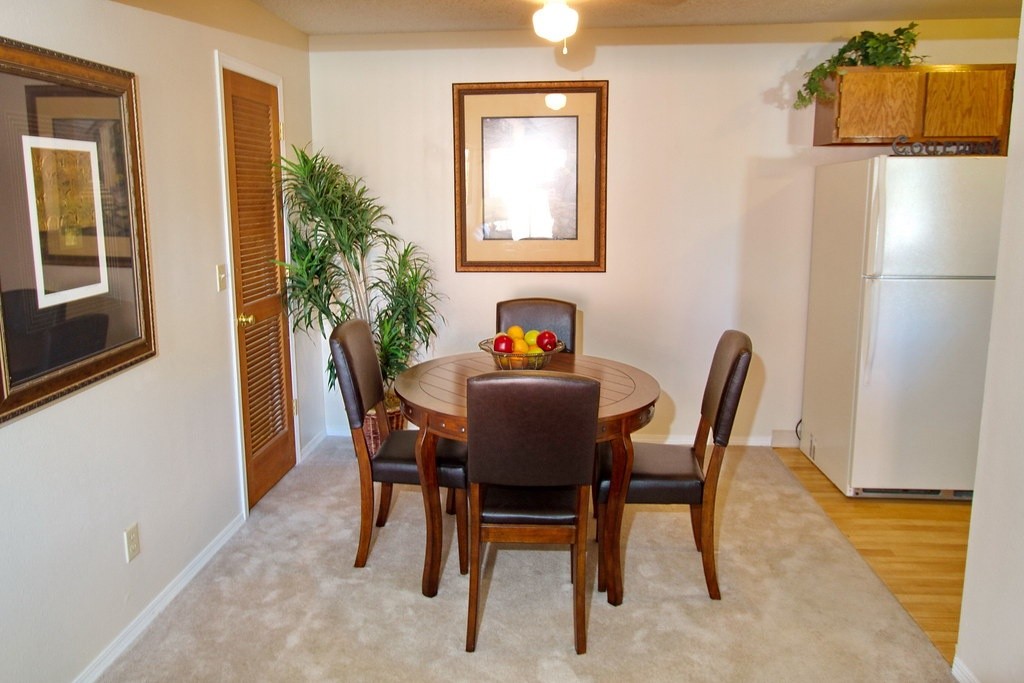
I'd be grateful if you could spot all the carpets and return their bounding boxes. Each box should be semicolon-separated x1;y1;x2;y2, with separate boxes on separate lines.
95;435;961;683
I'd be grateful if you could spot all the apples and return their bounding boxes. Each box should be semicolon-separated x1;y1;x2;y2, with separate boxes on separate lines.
536;331;556;351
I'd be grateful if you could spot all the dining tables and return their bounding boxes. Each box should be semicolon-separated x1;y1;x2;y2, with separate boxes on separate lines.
394;349;660;609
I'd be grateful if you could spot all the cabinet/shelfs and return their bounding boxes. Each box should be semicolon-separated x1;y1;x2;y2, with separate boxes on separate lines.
814;62;1016;144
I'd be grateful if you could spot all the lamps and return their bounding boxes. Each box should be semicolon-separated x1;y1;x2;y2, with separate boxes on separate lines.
532;1;580;55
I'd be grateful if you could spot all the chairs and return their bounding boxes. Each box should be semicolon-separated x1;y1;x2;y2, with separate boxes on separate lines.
330;319;473;575
496;296;576;353
465;372;601;657
591;329;753;607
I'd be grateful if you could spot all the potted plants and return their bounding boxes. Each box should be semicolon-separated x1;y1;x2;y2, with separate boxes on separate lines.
270;139;451;460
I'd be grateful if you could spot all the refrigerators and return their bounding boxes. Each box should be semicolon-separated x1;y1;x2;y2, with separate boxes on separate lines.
796;155;1011;504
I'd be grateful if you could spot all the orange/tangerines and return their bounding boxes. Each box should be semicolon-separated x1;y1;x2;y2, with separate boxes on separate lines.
494;325;545;369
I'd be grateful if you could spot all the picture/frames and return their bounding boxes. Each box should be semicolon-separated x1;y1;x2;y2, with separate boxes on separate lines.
0;36;157;425
451;79;609;274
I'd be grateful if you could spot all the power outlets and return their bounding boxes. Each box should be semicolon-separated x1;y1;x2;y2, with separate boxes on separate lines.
122;522;141;564
